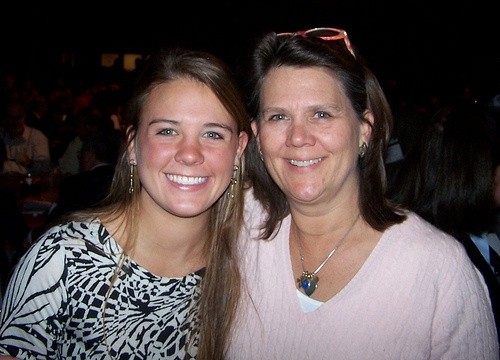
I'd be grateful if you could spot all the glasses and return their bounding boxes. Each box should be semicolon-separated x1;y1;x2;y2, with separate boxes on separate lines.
276;27;356;59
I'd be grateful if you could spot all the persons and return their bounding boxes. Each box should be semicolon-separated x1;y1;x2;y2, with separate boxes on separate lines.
219;27;500;359
0;49;249;360
1;98;122;299
384;108;500;338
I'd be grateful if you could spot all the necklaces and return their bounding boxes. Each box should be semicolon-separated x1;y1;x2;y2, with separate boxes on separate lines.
293;209;363;297
